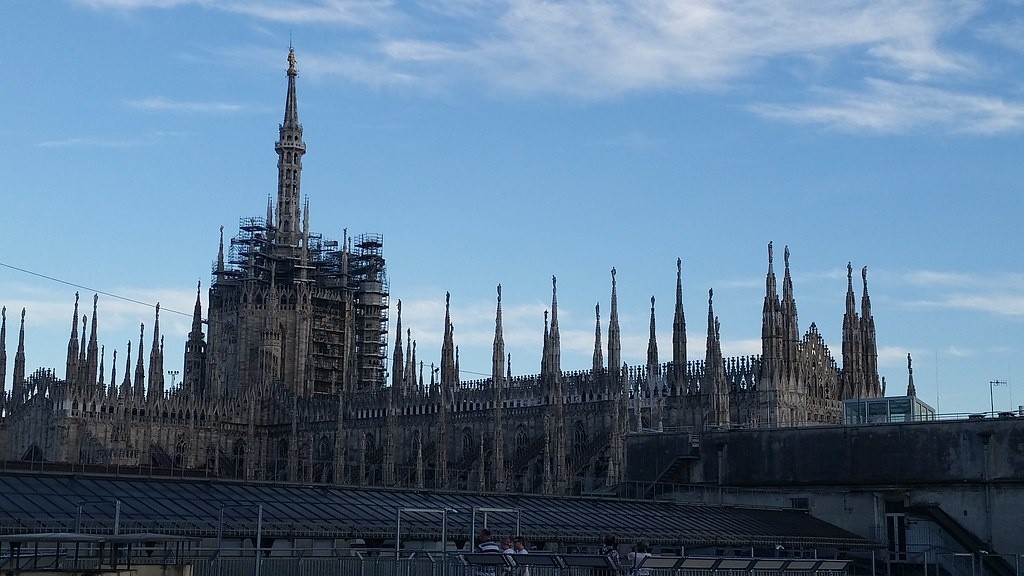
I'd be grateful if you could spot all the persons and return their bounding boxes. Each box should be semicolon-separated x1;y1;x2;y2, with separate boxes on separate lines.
500;537;517;575
594;535;620;576
475;529;499;576
513;536;529;576
625;538;651;576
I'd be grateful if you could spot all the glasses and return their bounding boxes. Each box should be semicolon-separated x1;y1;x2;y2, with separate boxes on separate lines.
514;541;520;544
